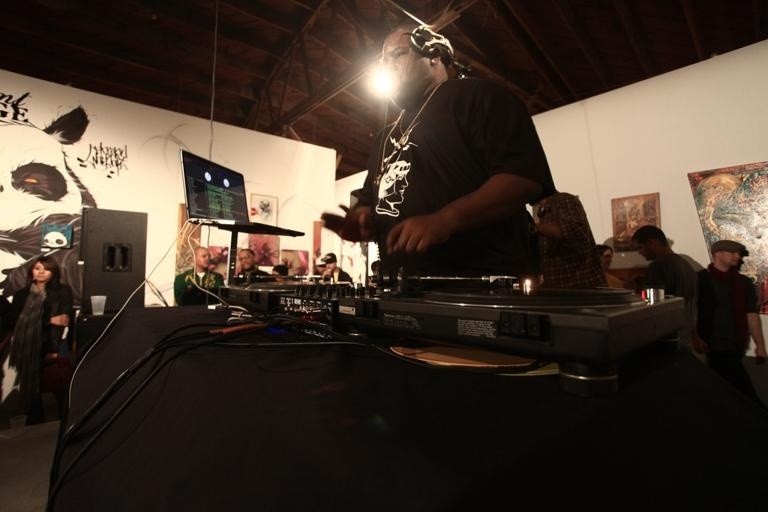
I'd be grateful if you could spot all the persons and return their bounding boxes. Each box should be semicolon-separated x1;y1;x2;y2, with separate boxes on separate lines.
695;239;768;361
320;22;553;294
310;254;326;282
271;265;288;282
595;243;626;291
8;254;74;426
174;246;225;306
231;249;277;283
320;253;353;283
622;224;693;298
533;188;610;289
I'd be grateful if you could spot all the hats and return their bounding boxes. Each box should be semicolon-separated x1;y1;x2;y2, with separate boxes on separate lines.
711;240;744;252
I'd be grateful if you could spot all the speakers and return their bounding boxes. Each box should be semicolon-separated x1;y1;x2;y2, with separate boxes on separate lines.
83;208;147;313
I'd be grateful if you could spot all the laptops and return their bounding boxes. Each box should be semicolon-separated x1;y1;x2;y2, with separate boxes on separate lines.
181;149;304;237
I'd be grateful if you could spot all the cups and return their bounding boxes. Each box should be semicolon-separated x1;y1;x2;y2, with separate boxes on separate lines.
90;295;107;316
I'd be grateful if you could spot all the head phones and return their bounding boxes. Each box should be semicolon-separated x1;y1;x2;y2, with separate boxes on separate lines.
411;25;455;57
